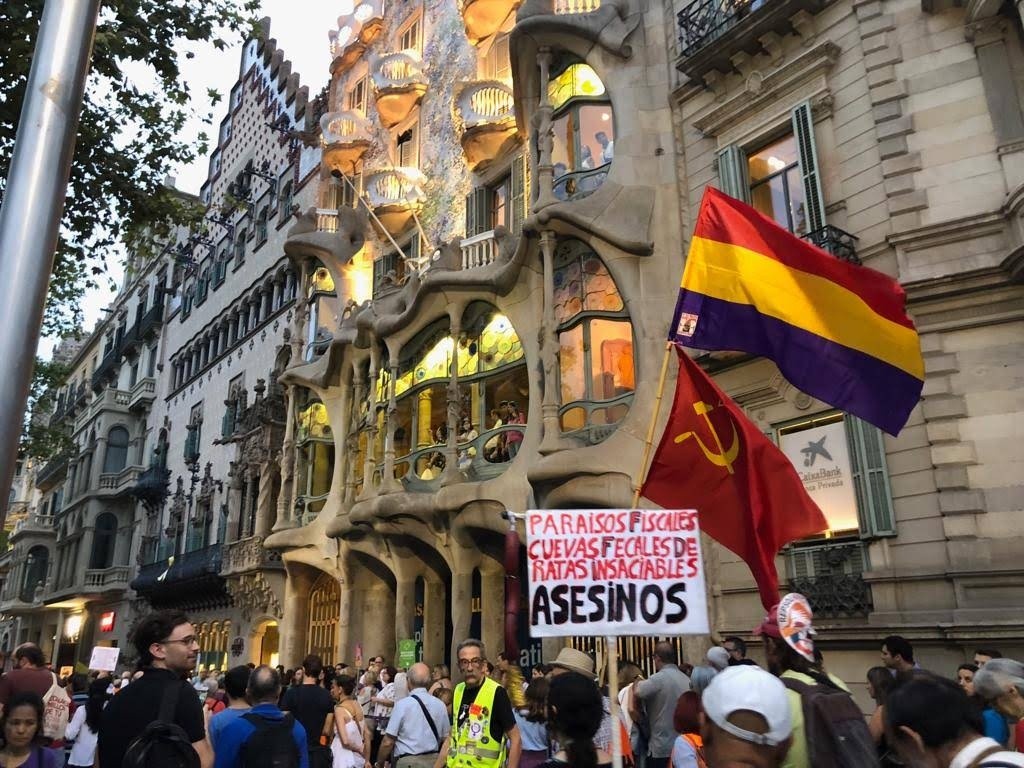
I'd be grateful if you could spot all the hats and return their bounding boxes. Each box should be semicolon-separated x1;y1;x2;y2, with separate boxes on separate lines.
549;647;596;677
707;647;730;670
701;665;793;746
752;604;783;638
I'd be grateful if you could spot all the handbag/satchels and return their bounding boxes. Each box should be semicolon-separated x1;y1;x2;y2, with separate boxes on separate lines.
120;720;202;768
40;671;72;740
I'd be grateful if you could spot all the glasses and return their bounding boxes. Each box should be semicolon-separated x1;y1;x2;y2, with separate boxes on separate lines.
461;657;481;667
651;654;662;658
159;637;199;646
724;648;737;651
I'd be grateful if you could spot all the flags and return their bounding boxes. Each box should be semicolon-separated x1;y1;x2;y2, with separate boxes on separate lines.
642;342;829;612
667;185;926;437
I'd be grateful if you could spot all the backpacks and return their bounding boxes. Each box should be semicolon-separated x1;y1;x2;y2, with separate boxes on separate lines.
778;671;880;768
236;712;298;768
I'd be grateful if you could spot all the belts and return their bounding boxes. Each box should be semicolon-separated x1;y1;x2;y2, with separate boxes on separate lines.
397;751;439;759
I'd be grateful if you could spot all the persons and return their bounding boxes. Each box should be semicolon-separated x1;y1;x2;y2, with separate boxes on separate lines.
424;401;528;480
553;132;613;201
0;603;1024;768
